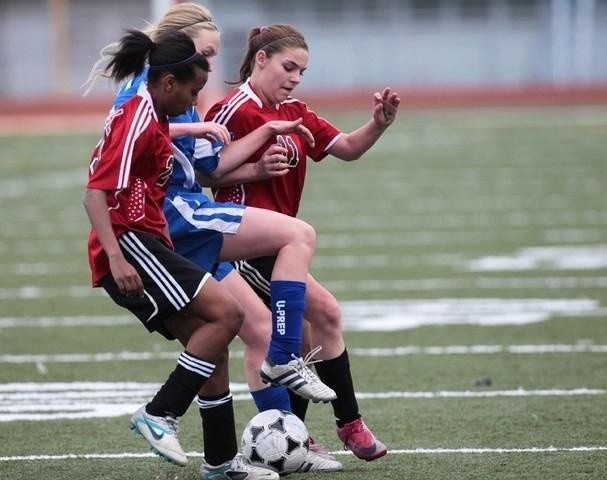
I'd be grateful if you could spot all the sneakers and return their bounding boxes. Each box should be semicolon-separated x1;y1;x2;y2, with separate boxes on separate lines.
296;436;343;472
130;402;188;467
199;453;281;480
261;345;338;403
336;414;387;462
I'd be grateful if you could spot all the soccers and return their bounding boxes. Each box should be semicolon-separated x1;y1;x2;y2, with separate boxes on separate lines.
241;409;309;473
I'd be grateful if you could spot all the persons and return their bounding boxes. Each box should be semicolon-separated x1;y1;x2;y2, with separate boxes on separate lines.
84;1;346;471
83;25;281;480
202;22;403;462
